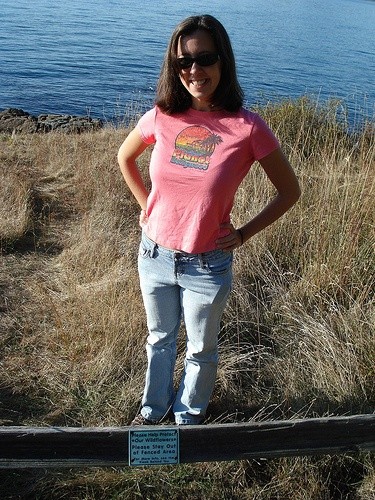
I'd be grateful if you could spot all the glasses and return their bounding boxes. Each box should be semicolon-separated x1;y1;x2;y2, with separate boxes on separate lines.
174;52;220;70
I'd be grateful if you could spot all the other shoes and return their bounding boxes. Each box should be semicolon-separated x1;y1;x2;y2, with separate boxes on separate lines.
130;414;157;426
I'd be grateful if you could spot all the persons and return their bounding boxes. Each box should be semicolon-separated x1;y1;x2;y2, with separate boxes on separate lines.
117;14;301;425
